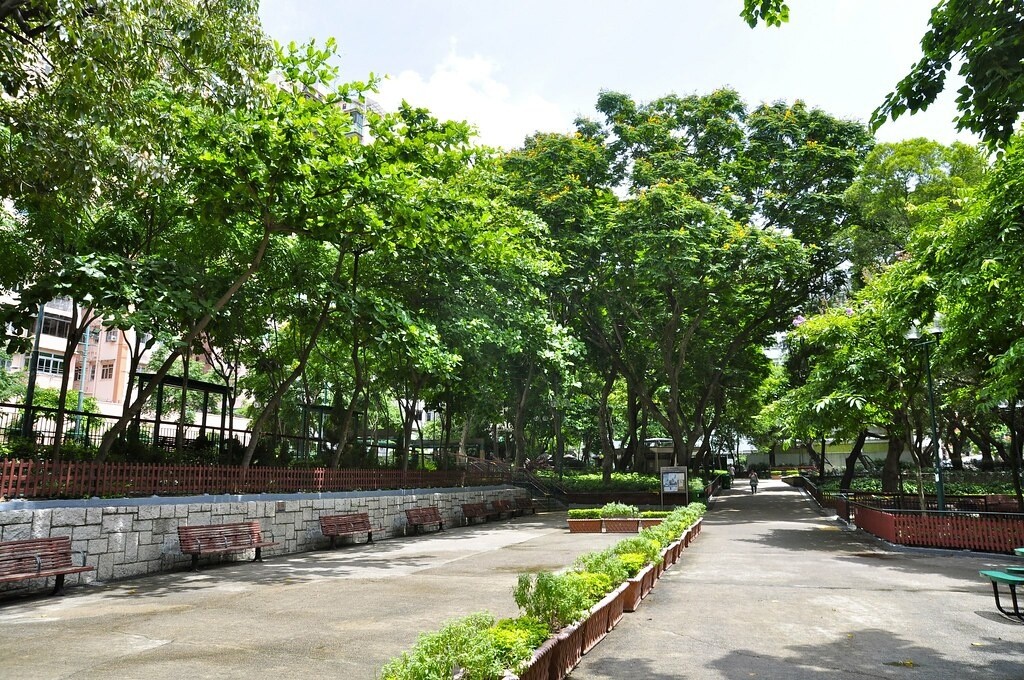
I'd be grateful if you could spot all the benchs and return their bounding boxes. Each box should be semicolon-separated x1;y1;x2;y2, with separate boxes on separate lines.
0;535;95;595
460;503;499;526
513;496;541;516
493;500;523;519
977;568;1024;622
319;512;387;551
404;507;450;536
177;520;281;572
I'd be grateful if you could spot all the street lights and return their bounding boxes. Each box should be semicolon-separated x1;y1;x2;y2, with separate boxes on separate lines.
902;316;947;519
72;325;102;443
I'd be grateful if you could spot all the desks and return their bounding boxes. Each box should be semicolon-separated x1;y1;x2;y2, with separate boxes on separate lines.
1014;548;1024;556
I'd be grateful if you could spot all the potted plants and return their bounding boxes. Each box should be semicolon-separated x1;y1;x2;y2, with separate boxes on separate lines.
579;544;647;610
639;529;677;570
567;549;630;631
645;525;681;564
602;500;641;533
666;513;691;547
622;538;664;588
683;502;707;534
676;505;700;538
375;609;558;680
671;508;696;543
613;539;658;599
655;521;683;558
632;529;669;578
640;510;673;529
512;567;591;680
559;570;619;654
567;508;602;533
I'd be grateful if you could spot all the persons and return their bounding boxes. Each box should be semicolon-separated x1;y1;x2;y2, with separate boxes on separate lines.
747;470;759;495
728;463;735;485
